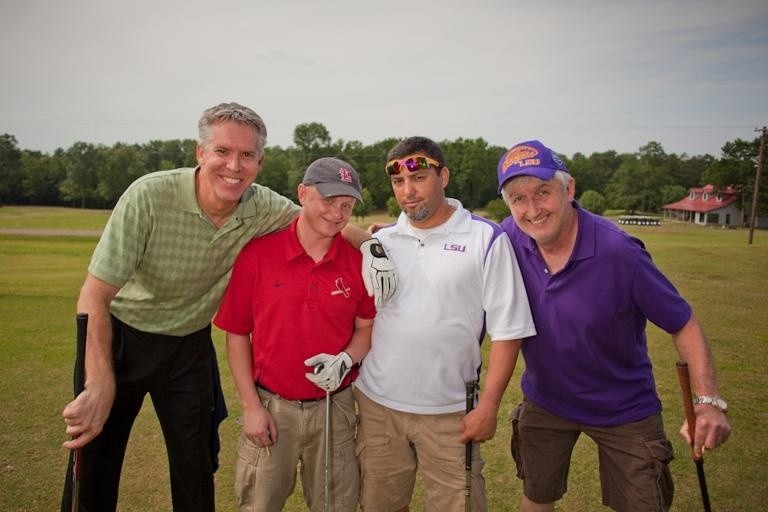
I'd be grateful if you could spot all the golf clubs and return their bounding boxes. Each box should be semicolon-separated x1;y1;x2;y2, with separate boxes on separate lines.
314;363;330;512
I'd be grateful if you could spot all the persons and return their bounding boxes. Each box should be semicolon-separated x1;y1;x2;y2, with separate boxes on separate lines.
352;135;537;512
496;140;733;512
60;103;401;512
213;156;376;512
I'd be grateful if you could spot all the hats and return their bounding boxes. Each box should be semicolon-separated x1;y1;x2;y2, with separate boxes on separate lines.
302;157;368;208
496;140;570;196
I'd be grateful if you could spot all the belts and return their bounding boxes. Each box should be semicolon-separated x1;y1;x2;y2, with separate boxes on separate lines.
254;380;353;403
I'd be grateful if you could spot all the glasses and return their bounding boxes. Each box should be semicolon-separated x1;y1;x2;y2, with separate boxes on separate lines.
383;157;440;175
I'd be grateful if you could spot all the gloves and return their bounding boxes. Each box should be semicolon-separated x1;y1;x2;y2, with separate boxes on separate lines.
359;238;401;309
303;350;355;392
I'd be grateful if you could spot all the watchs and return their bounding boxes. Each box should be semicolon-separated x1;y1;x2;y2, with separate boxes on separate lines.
695;395;729;413
